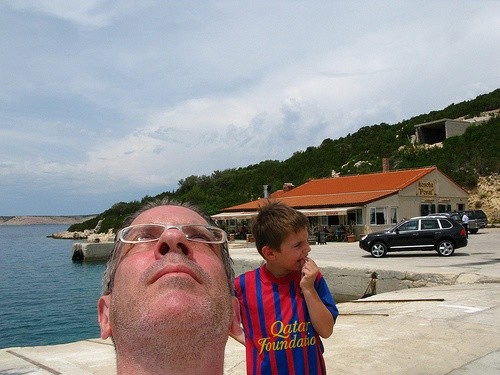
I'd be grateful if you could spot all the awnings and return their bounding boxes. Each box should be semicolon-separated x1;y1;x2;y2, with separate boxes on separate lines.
209;205;364;220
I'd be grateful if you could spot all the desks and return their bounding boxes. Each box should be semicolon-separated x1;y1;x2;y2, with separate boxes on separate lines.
325;230;349;241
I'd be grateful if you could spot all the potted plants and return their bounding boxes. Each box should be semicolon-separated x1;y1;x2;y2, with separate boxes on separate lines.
347;220;356;242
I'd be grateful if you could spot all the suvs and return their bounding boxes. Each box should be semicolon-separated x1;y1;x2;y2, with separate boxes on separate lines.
359;210;488;258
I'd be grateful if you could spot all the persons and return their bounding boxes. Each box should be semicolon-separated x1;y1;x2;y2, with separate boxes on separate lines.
97;197;243;375
233;220;353;242
228;199;339;375
462;212;469;239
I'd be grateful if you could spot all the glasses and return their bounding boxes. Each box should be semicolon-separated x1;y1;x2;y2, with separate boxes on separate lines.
113;223;228;251
